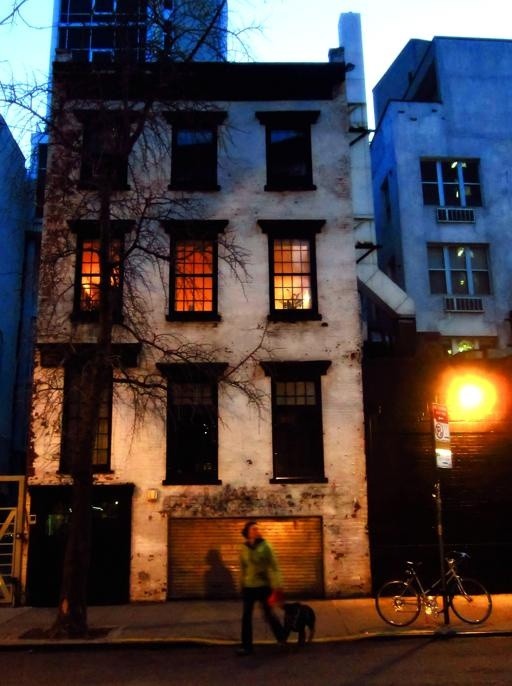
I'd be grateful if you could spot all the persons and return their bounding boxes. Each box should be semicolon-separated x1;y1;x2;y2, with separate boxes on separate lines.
233;521;288;655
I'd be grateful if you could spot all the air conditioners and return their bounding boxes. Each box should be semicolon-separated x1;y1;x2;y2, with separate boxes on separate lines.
280;601;316;645
